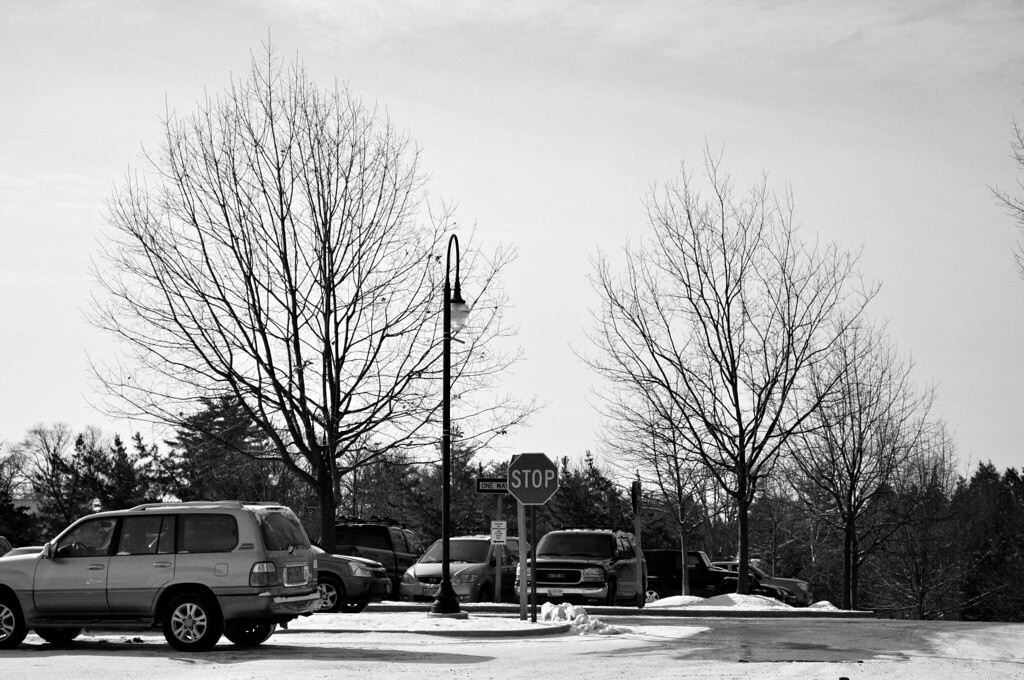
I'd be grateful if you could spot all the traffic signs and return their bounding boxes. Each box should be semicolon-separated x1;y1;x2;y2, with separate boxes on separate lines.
475;477;509;494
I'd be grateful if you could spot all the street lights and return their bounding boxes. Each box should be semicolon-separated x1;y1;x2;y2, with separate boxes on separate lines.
429;232;469;614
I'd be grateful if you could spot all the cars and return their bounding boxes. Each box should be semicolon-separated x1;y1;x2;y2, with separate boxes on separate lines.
308;544;393;612
711;560;812;605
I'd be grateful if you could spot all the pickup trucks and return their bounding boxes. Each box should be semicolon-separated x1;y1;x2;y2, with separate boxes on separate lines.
333;523;424;597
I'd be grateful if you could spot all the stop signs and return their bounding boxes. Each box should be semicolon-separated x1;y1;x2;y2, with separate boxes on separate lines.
507;453;558;505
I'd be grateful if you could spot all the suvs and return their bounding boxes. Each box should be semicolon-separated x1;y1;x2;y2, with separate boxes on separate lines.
513;528;648;606
638;547;758;605
400;533;532;602
0;498;320;655
